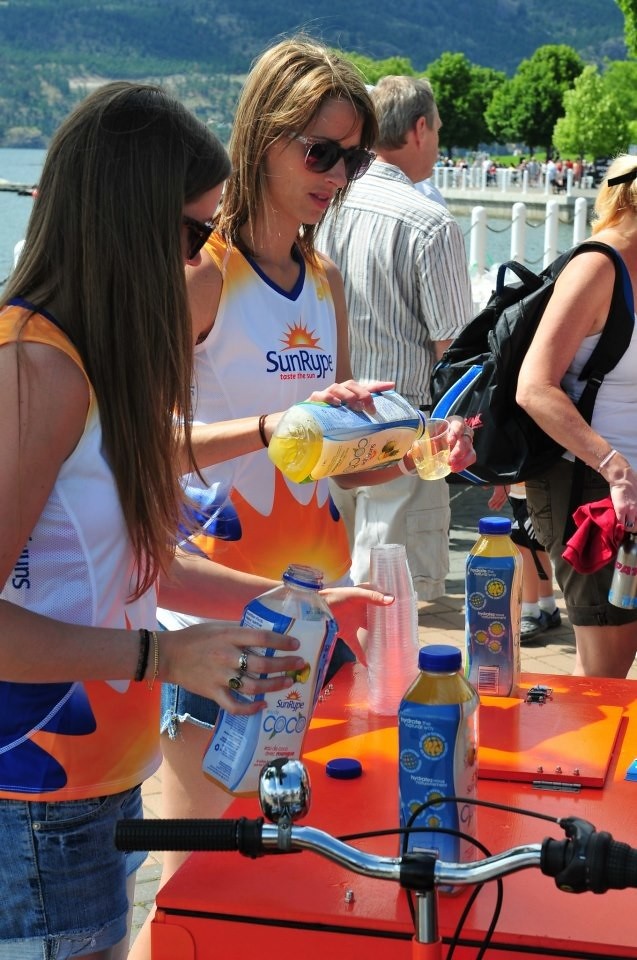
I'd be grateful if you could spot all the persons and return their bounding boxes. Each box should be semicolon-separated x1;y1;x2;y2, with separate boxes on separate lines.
0;79;396;960
435;151;586;193
515;153;637;679
307;75;474;599
129;38;476;959
482;480;563;641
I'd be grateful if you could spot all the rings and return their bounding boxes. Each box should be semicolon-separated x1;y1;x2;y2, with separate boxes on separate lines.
227;673;243;690
626;521;634;528
238;652;248;672
462;433;474;442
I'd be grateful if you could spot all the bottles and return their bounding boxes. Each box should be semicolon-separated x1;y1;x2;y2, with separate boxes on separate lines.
398;645;480;894
608;533;637;609
202;564;339;797
268;389;428;484
464;517;524;694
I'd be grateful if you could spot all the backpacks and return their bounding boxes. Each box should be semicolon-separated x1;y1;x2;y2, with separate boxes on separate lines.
429;242;635;486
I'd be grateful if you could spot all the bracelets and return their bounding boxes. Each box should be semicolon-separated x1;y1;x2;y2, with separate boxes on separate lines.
148;631;159;691
258;414;270;448
134;629;150;681
397;457;419;477
596;449;618;473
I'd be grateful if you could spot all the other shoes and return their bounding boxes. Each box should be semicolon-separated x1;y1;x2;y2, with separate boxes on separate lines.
520;610;548;640
541;607;563;630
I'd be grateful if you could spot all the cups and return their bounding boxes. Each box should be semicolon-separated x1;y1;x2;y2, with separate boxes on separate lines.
367;544;420;715
412;418;451;480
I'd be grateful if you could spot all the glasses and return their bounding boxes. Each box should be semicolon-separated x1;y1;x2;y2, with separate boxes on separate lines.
182;214;221;259
289;132;377;180
